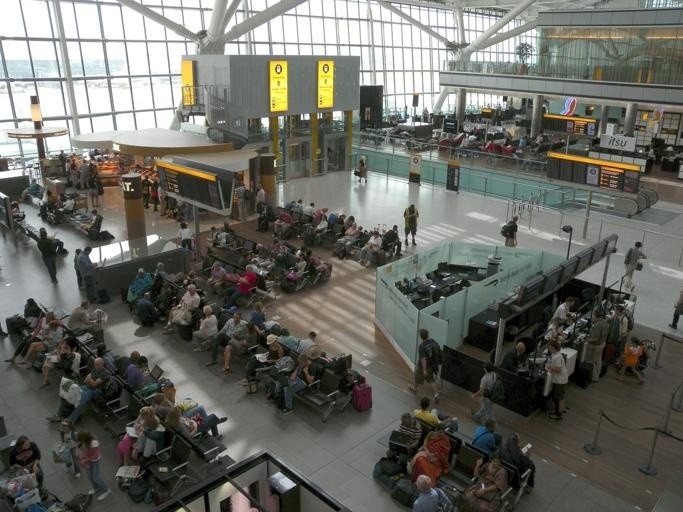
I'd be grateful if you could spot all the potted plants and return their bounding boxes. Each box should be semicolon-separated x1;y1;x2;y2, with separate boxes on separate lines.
516;43;533;75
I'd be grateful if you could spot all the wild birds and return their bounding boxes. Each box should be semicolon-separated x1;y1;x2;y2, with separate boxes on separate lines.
148;365;164;381
438;262;452;277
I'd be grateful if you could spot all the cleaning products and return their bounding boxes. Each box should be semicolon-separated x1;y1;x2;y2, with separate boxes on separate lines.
613;339;656;376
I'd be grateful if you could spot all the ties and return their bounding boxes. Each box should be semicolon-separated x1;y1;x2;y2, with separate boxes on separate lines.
529;359;546;371
613;294;625;304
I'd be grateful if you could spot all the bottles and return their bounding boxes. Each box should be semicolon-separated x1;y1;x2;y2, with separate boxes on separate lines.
267;335;277;345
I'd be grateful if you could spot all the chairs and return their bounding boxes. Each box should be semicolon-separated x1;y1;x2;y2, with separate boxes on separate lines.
280;407;295;415
4;441;43;486
46;415;71;427
373;415;531;510
88;484;111;501
219;366;231;376
18;298;227;498
207;206;397;306
127;269;365;421
193;345;204;352
434;129;568;171
0;186;103;255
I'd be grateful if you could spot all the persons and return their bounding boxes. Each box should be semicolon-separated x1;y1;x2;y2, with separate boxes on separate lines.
649;146;654;155
378;106;543;162
9;435;43;489
669;290;683;329
0;299;227;500
404;204;419;246
11;201;25;221
624;242;647;292
142;176;168;215
38;228;69;283
60;149;118;207
386;397;535;512
472;363;497;426
408;329;442;401
505;215;519;246
81;208;101;236
359;157;367;183
235;183;275;232
37;191;74;225
75;246;98;300
502;298;644;420
667;144;673;151
273;198;404;267
129;221;334;414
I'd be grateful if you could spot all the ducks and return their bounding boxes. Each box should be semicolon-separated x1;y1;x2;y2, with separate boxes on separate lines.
172;303;192;340
377;250;385;265
576;362;593;388
353;377;372;411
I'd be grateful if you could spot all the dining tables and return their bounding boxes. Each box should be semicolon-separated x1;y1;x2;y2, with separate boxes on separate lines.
516;43;533;75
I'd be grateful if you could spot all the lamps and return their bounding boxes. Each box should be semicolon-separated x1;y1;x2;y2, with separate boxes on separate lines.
434;129;568;171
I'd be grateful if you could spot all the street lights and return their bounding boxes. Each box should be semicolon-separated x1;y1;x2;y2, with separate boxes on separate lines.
0;470;92;512
374;457;418;507
115;466;153;503
172;308;192;326
437;492;459;512
59;372;93;408
239;377;261;394
490;378;505;402
53;447;70;463
431;343;446;374
